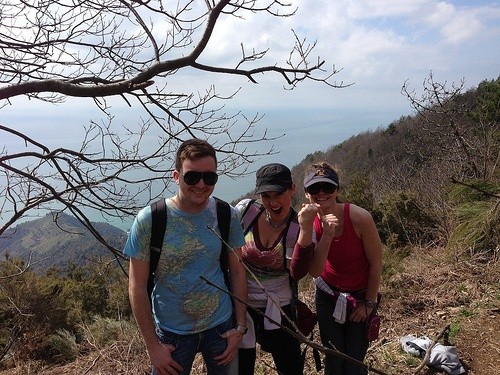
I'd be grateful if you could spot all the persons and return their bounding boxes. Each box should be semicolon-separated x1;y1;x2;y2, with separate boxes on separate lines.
303;161;383;375
123;138;248;375
237;162;318;375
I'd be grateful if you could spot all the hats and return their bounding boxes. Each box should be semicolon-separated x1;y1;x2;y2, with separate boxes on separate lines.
254;163;292;195
303;162;340;187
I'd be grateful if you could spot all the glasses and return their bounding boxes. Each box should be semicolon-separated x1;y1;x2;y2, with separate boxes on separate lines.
183;171;218;186
304;185;338;195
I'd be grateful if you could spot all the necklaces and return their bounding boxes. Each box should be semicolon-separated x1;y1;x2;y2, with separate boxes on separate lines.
265;208;291;228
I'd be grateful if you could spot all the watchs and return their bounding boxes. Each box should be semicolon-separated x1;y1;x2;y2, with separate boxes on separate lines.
235;324;248;336
365;299;376;308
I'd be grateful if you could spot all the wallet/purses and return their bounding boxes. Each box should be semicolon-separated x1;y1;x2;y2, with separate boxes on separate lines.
365;302;380;340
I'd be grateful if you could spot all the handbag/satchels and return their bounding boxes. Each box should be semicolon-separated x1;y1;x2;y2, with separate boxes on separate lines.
286;279;318;344
